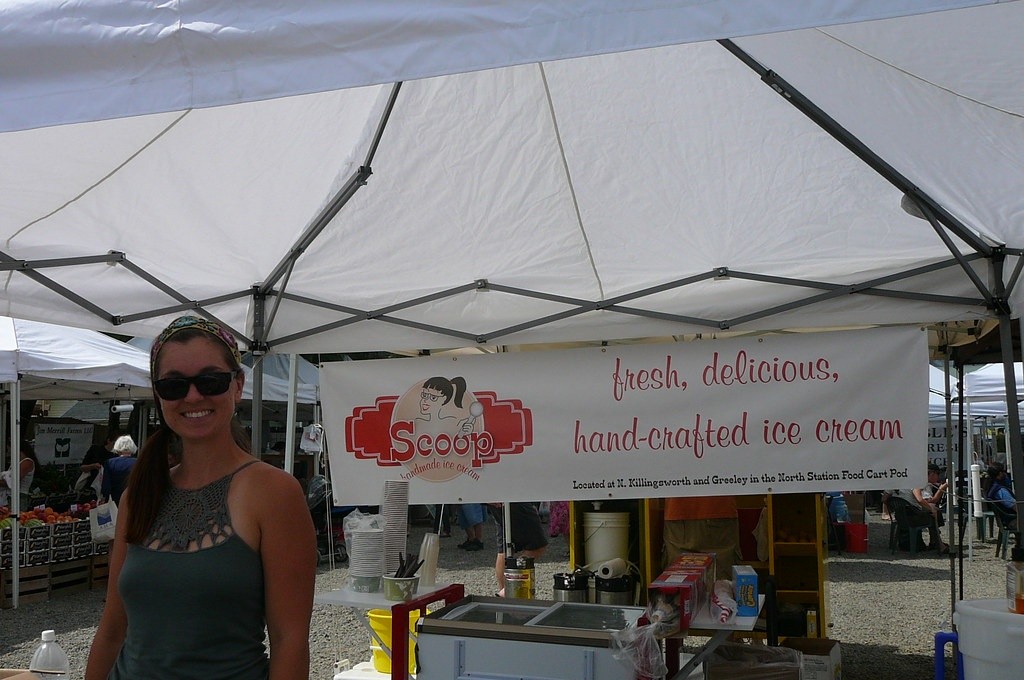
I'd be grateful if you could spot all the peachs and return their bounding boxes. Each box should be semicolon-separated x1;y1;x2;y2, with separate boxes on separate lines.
0;507;75;525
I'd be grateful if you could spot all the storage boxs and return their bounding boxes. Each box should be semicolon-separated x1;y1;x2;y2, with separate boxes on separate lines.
652;549;840;679
0;519;108;568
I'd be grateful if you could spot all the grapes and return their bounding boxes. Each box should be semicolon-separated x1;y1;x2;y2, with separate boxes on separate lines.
70;511;91;519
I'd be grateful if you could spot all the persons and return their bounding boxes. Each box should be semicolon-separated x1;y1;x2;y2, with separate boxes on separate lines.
79;432;119;503
101;435;138;508
487;503;548;598
264;441;285;470
84;316;317;680
0;441;41;512
881;464;1024;555
457;504;487;551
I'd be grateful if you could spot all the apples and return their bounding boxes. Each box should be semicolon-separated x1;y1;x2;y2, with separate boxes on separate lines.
76;500;97;511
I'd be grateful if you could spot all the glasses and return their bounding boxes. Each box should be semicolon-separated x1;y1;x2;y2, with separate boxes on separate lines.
153;370;239;401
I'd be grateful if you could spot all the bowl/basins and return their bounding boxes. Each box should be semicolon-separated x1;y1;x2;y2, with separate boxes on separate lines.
391;572;420;594
383;575;414;601
349;528;383;594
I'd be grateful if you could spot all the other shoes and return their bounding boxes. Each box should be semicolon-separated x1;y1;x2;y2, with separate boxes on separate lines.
938;546;957;557
929;541;948;550
457;538;474;549
466;538;484;551
443;531;451;537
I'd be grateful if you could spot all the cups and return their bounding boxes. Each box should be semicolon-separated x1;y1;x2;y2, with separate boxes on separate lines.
383;480;440;585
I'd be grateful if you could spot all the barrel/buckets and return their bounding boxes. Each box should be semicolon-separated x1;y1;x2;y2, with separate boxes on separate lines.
367;609;431;674
582;512;629;566
846;523;868;553
953;599;1024;680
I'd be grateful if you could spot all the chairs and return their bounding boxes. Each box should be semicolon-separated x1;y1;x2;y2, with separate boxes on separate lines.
884;489;1018;559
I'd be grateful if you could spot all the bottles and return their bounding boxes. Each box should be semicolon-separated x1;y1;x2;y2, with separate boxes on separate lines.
836;501;848;522
30;630;70;680
1005;547;1024;615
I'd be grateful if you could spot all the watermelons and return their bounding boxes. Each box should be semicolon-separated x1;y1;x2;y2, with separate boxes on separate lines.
24;518;43;527
0;517;13;528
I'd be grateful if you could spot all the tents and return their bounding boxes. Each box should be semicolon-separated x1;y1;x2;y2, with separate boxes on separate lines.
237;352;320;421
0;330;320;610
0;0;1024;680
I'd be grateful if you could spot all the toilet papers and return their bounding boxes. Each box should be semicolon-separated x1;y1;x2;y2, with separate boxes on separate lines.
597;557;628;578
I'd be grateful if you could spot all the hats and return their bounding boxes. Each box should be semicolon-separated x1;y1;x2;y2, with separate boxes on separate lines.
928;463;940;471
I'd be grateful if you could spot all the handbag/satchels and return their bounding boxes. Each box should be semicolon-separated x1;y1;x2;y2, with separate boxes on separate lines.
90;492;119;544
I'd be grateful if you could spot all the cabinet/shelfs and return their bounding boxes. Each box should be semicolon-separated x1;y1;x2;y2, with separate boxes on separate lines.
570;493;825;640
